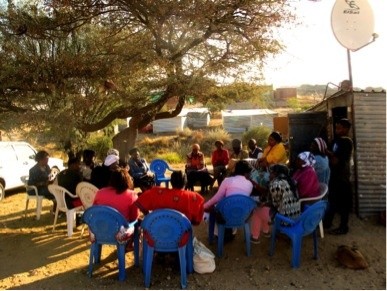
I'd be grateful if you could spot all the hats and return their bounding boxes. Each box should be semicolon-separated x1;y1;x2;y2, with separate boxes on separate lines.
298;151;315;167
192;144;200;150
315;138;328;151
104;154;119;166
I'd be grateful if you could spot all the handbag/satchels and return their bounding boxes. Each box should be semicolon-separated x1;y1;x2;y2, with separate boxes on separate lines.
192;236;216;274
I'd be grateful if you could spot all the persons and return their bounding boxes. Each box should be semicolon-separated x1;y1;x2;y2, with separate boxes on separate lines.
27;119;354;265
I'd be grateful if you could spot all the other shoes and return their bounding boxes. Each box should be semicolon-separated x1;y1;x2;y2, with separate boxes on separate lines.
263;233;270;238
250;236;261;243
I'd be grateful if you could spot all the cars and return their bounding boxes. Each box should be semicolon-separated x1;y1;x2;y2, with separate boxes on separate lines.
0;141;66;202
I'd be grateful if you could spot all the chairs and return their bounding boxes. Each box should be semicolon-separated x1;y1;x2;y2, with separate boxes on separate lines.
20;175;46;221
76;182;100;240
82;205;140;280
268;200;327;268
142;208;194;290
208;194;257;258
297;182;328;238
47;184;85;237
150;159;177;188
